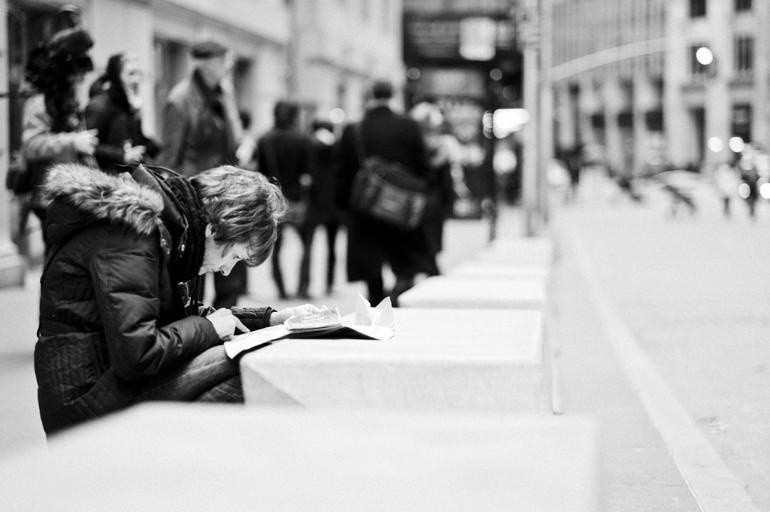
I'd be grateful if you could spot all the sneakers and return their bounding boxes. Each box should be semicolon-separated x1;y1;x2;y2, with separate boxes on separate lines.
276;278;413;306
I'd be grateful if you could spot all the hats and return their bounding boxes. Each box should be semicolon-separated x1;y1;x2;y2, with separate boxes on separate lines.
190;40;228;59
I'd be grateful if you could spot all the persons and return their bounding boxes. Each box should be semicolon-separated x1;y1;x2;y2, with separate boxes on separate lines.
1;23;523;312
32;155;328;432
561;131;763;218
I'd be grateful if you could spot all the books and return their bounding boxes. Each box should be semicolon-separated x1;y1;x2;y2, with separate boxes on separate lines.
222;293;382;363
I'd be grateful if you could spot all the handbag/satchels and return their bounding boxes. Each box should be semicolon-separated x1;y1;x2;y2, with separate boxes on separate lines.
356;161;428;228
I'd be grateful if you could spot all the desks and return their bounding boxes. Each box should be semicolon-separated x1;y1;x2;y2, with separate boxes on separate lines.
397;236;556;306
237;304;542;416
0;399;602;512
